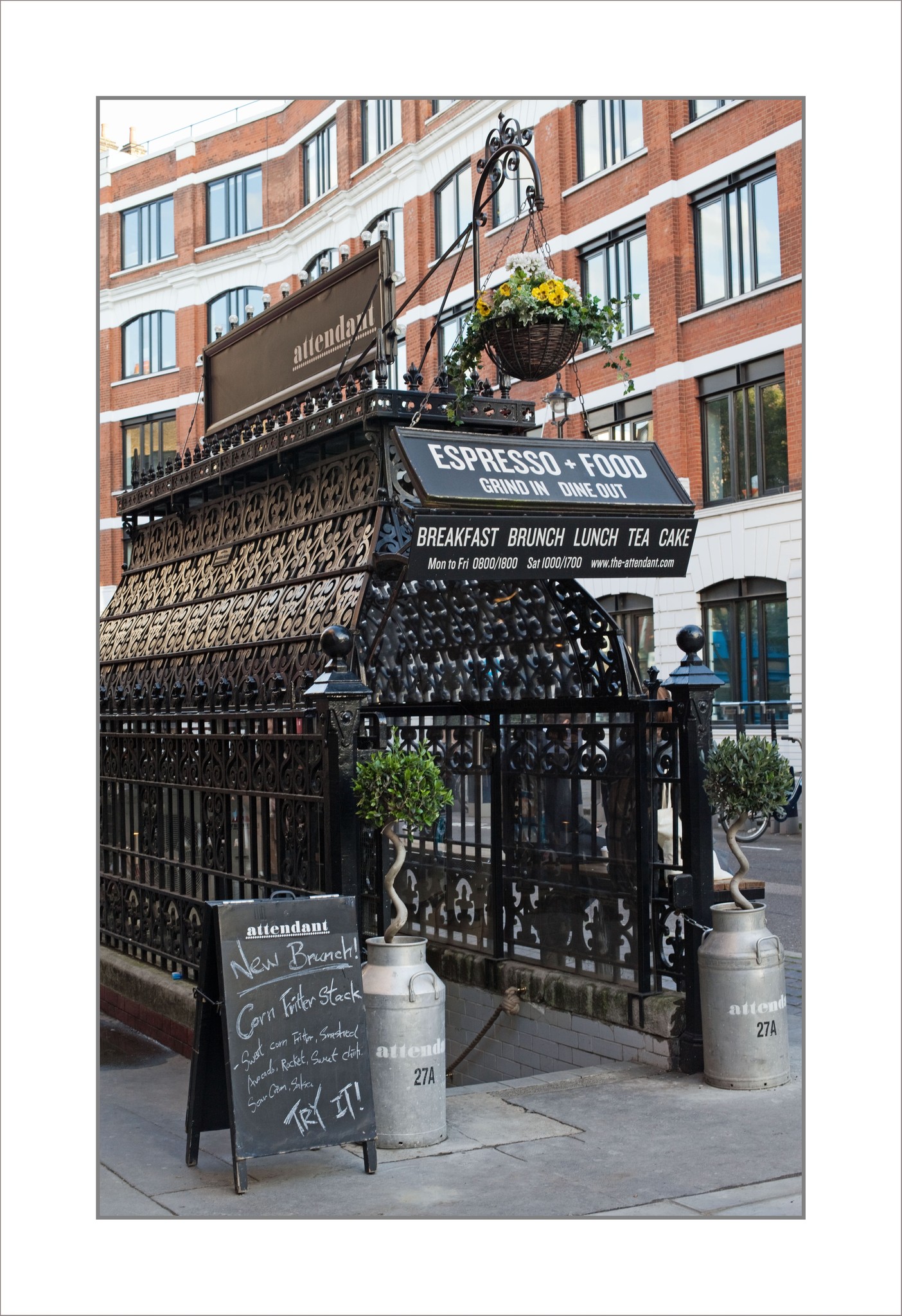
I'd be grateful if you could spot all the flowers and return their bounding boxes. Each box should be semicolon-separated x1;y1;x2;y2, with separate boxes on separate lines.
437;251;639;431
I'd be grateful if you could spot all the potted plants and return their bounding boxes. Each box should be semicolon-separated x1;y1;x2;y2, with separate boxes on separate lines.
698;728;796;1091
351;727;450;1151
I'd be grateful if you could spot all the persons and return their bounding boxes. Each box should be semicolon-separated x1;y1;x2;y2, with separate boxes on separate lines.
506;686;609;953
598;679;731;885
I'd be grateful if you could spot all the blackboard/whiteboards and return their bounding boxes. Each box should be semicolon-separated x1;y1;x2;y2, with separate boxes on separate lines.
183;890;378;1163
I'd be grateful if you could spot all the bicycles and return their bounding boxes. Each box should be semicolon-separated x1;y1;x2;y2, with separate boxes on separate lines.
719;737;801;843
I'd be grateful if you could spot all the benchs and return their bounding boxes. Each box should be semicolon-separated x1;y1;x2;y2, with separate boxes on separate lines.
389;840;765;910
